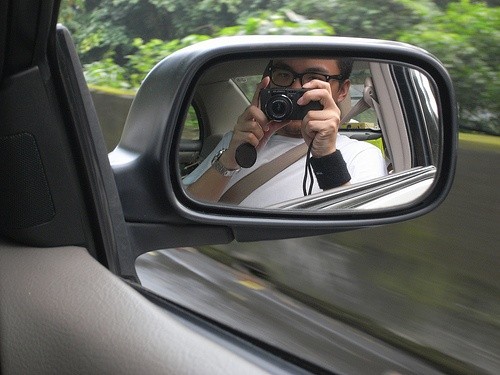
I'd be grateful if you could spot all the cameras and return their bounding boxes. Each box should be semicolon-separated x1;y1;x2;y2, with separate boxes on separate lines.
260;86;322;122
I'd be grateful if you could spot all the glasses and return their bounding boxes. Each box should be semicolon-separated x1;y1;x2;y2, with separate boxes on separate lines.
267;59;344;90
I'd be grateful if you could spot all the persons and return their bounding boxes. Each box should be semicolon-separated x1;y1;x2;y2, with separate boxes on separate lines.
181;56;385;210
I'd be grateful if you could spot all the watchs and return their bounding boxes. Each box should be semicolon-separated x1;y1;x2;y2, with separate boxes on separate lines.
211;148;241;177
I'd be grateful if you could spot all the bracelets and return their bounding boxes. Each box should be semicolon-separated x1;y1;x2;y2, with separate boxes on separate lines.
309;149;351;189
